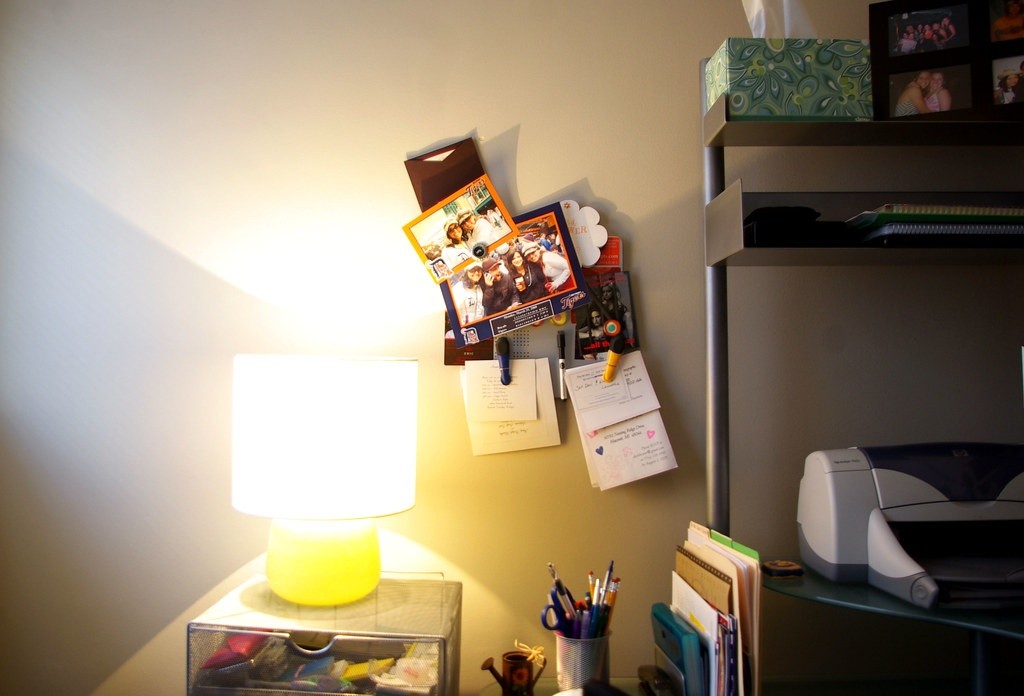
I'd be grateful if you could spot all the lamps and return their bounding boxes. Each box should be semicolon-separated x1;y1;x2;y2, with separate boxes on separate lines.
231;350;420;605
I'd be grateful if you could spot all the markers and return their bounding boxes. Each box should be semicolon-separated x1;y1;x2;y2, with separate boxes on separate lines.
556;331;568;401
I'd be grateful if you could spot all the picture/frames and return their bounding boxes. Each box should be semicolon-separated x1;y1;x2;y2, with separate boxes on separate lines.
868;0;1024;121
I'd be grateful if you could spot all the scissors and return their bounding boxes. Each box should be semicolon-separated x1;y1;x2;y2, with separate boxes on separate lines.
541;585;576;637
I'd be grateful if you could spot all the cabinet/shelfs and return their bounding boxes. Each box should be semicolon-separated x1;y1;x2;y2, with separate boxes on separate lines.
698;54;1024;538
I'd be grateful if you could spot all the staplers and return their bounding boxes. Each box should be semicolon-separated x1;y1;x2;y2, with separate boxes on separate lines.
636;664;676;696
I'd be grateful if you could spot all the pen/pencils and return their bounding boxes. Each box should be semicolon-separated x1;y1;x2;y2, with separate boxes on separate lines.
547;559;622;682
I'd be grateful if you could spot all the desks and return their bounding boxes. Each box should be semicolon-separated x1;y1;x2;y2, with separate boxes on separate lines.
188;551;462;696
758;555;1024;696
479;676;645;696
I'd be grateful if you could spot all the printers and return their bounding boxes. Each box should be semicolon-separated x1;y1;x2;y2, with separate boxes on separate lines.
797;441;1024;608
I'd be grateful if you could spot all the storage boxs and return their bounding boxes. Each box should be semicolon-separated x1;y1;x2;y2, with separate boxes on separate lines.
705;38;874;122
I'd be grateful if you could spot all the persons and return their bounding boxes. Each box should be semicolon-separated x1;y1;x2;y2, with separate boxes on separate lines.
452;231;571;326
579;281;627;340
424;207;513;267
887;4;1024;117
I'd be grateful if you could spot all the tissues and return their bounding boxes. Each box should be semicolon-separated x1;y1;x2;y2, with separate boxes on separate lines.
704;0;873;122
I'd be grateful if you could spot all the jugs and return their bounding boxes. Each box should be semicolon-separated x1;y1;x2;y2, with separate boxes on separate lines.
481;651;548;696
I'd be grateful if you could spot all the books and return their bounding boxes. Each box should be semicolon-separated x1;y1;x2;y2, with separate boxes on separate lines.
278;657;393;681
651;602;705;696
